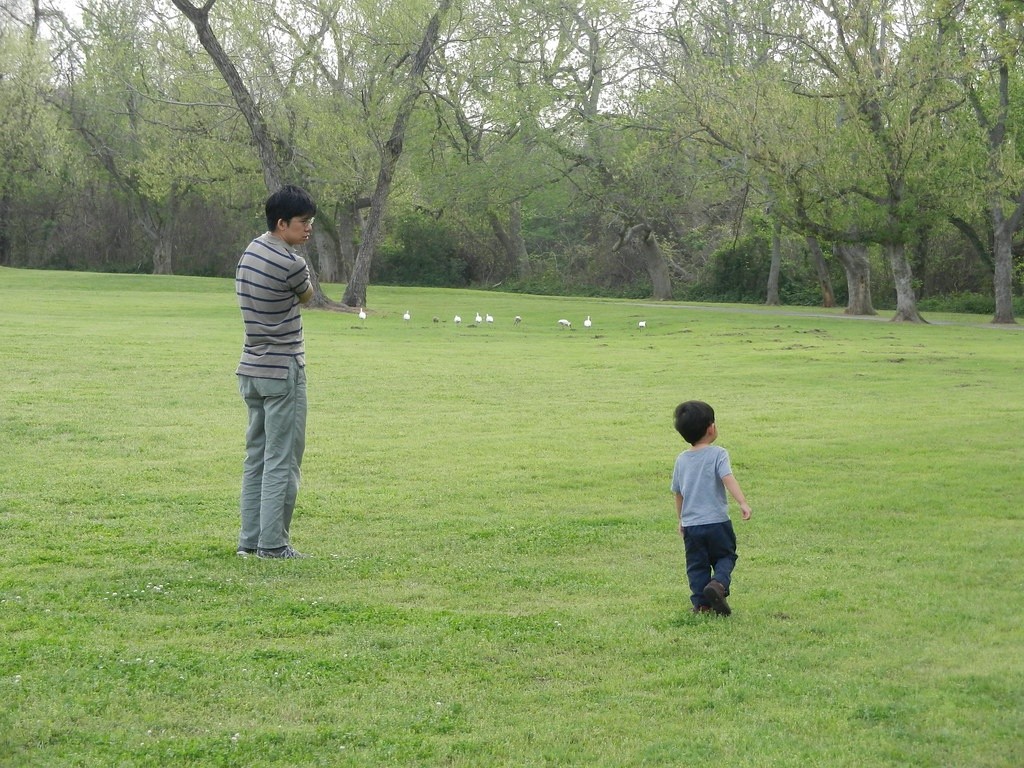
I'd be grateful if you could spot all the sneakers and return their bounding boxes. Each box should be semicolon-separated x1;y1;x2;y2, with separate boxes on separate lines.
236;546;257;555
702;579;731;615
256;546;311;559
693;604;721;614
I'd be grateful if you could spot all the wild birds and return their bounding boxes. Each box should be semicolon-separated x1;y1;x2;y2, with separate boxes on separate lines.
403;310;411;322
453;315;462;324
475;312;483;323
358;307;366;320
512;316;522;326
583;315;592;329
558;319;572;330
432;316;440;323
486;314;494;322
638;320;646;332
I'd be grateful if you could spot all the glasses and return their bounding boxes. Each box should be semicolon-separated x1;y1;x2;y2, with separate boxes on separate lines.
288;217;315;225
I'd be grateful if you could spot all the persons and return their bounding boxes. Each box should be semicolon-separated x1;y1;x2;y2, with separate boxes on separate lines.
235;186;316;560
671;401;752;617
